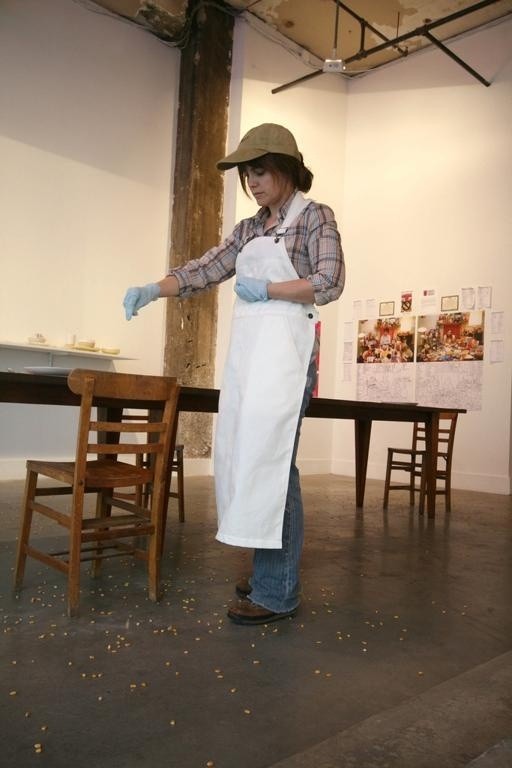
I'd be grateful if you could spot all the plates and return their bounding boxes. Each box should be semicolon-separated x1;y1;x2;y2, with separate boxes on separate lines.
23;366;74;376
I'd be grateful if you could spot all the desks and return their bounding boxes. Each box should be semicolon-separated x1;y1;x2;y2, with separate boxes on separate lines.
1;371;468;510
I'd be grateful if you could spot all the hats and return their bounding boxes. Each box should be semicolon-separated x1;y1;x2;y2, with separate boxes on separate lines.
215;123;303;171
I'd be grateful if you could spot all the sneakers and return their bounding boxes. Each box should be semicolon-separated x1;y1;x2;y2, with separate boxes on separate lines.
235;578;253;596
227;595;298;625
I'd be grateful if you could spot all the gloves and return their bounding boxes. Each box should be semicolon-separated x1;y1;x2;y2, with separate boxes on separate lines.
233;274;271;303
123;282;162;321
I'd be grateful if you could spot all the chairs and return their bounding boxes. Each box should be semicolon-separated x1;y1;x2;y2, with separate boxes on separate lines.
10;366;187;607
382;413;457;515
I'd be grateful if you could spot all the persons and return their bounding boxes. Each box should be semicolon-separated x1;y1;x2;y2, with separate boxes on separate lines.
120;121;346;627
